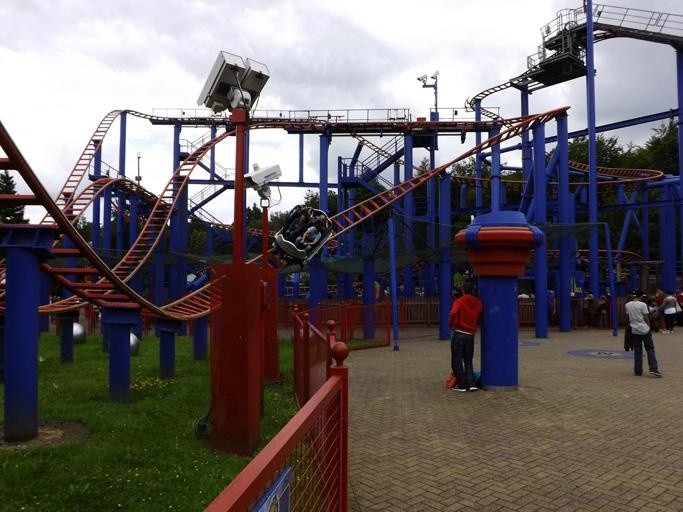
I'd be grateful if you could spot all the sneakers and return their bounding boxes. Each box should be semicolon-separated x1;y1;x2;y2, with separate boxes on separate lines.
451;385;478;391
662;330;673;333
650;369;661;376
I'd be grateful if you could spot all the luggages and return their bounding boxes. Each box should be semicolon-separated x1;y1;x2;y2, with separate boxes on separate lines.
624;326;633;351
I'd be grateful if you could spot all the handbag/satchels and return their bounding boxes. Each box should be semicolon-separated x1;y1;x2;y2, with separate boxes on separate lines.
676;302;682;312
446;372;458;388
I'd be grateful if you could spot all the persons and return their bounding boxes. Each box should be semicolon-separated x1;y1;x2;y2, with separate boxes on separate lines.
578;293;596;327
286;208;328;252
625;288;661;377
641;287;683;333
598;286;611;329
448;282;483;391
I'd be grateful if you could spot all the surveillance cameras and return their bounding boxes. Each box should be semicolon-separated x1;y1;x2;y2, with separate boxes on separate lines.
417;74;427;81
243;164;282;189
227;58;270;111
431;70;439;78
197;51;245;114
